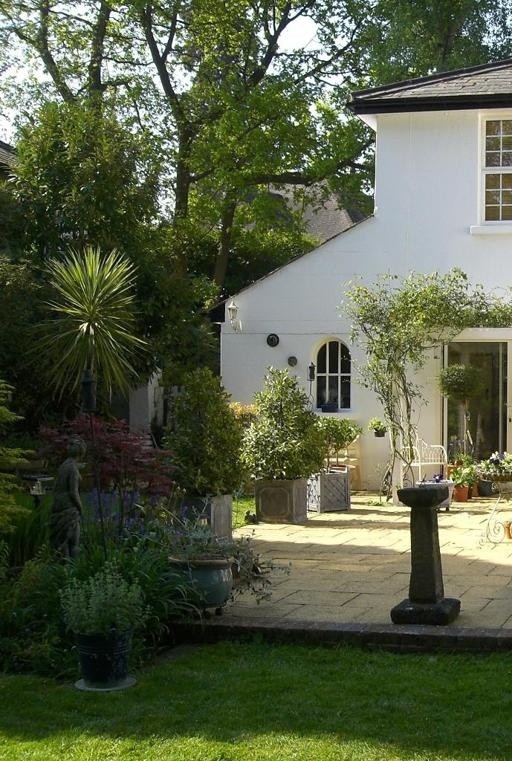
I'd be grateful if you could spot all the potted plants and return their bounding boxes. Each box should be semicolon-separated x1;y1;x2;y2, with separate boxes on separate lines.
368;416;387;437
434;362;512;546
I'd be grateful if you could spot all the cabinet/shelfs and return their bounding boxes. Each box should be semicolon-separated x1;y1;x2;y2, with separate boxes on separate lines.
391;424;448;506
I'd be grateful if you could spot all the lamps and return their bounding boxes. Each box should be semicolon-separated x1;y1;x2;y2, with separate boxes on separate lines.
226;301;242;334
307;362;317;401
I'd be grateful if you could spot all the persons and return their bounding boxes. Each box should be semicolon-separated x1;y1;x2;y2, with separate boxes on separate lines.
51;436;85;559
